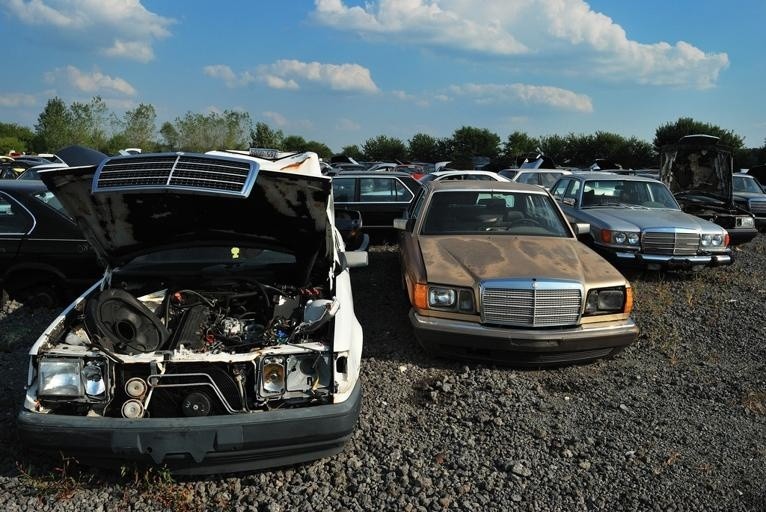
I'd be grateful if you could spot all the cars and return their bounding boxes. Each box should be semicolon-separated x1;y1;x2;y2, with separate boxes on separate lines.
392;178;641;371
16;153;376;481
548;172;734;280
0;179;104;308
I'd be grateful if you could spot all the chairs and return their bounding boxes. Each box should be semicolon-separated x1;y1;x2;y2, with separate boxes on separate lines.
505;211;526;225
